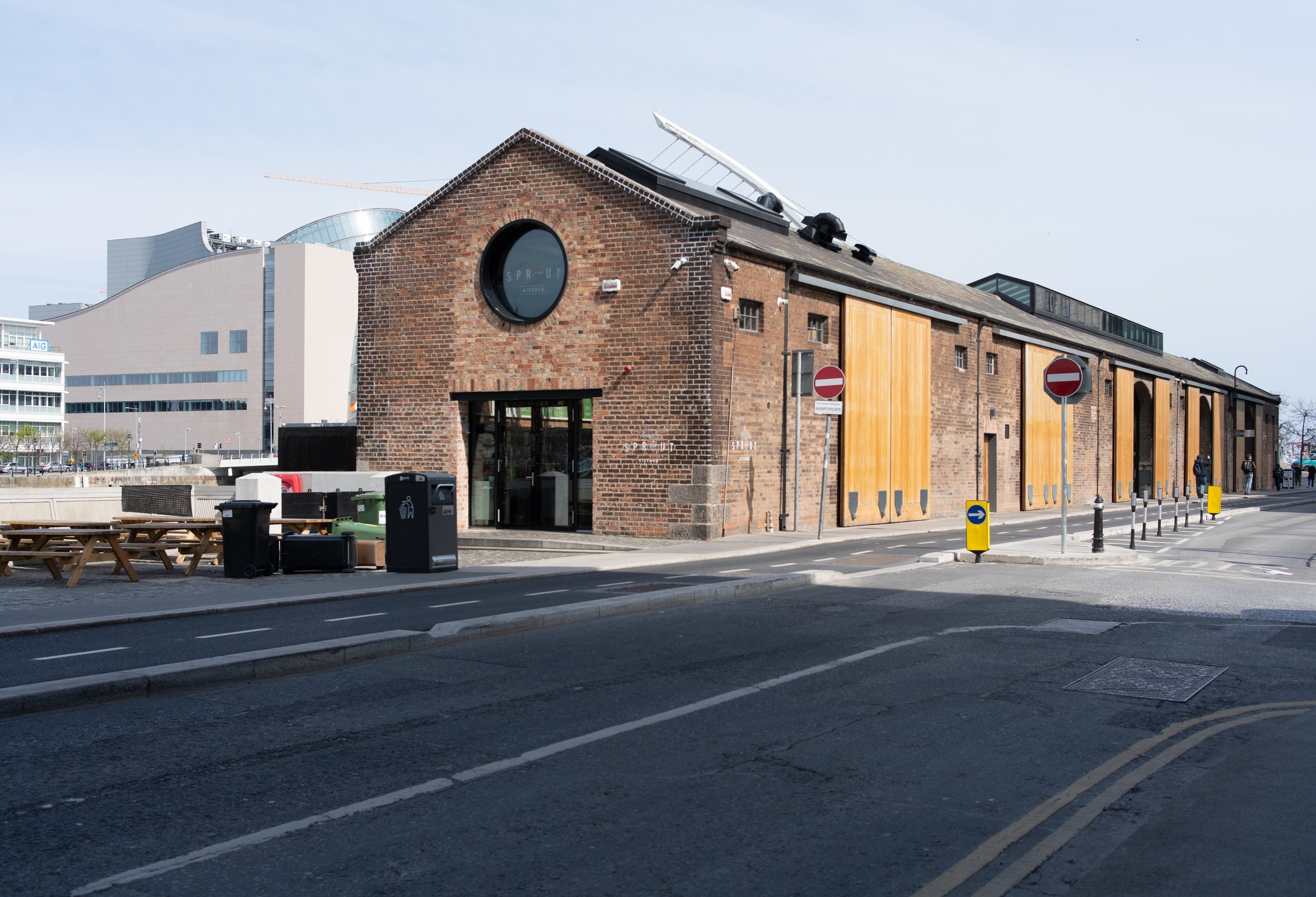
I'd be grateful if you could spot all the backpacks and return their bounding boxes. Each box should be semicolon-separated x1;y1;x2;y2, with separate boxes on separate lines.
1193;463;1204;475
1244;459;1252;472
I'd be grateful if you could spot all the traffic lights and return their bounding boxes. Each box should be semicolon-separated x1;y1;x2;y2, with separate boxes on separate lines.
136;443;139;448
213;443;217;449
219;443;222;449
195;449;198;453
197;442;201;449
82;450;87;457
154;451;157;455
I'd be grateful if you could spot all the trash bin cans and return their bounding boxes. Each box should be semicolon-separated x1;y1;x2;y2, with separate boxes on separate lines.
358;536;387;569
351;491;385;526
269;531;357;575
214;499;278;579
1282;469;1295;488
331;516;386;554
384;472;458;574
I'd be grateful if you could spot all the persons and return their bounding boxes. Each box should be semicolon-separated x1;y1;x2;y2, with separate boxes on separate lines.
41;461;44;464
1272;464;1284;491
85;461;91;471
1294;464;1301;487
67;460;68;464
1241;454;1256;495
80;462;83;471
181;454;185;465
1307;465;1316;488
1195;455;1211;499
156;460;160;467
164;460;167;466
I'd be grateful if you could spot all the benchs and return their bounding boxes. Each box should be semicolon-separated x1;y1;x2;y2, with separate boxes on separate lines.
0;530;284;565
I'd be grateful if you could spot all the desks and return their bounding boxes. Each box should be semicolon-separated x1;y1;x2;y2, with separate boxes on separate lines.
1;519;120;572
111;523;222;576
112;515;222;564
0;528;140;588
269;518;335;536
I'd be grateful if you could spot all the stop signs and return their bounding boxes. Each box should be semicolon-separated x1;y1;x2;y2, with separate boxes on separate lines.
1044;358;1082;398
813;366;846;399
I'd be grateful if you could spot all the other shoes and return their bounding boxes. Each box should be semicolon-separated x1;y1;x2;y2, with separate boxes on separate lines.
1311;487;1313;488
1307;485;1310;488
1247;493;1251;495
1198;492;1201;498
1294;485;1298;487
1277;489;1280;491
1198;496;1201;499
1244;492;1247;495
1299;483;1300;486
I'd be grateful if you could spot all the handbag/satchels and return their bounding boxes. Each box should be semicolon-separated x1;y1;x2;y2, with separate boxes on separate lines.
1280;478;1283;483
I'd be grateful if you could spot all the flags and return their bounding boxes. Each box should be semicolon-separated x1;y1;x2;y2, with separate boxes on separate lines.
349;401;357;412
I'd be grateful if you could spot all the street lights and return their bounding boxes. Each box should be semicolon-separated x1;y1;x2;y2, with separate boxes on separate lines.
71;428;78;464
185;428;191;459
263;395;272;456
32;438;38;475
125;405;140;468
235;432;240;457
127;434;132;469
96;381;105;470
274;405;286;428
258;438;261;458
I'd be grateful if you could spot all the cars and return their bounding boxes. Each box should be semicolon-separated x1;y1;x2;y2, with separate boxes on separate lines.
98;463;122;470
0;462;33;474
36;462;88;472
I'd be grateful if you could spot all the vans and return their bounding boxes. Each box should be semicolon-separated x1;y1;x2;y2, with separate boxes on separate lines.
1291;453;1316;471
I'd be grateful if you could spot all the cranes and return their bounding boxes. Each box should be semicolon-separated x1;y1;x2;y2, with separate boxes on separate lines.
261;170;439;197
